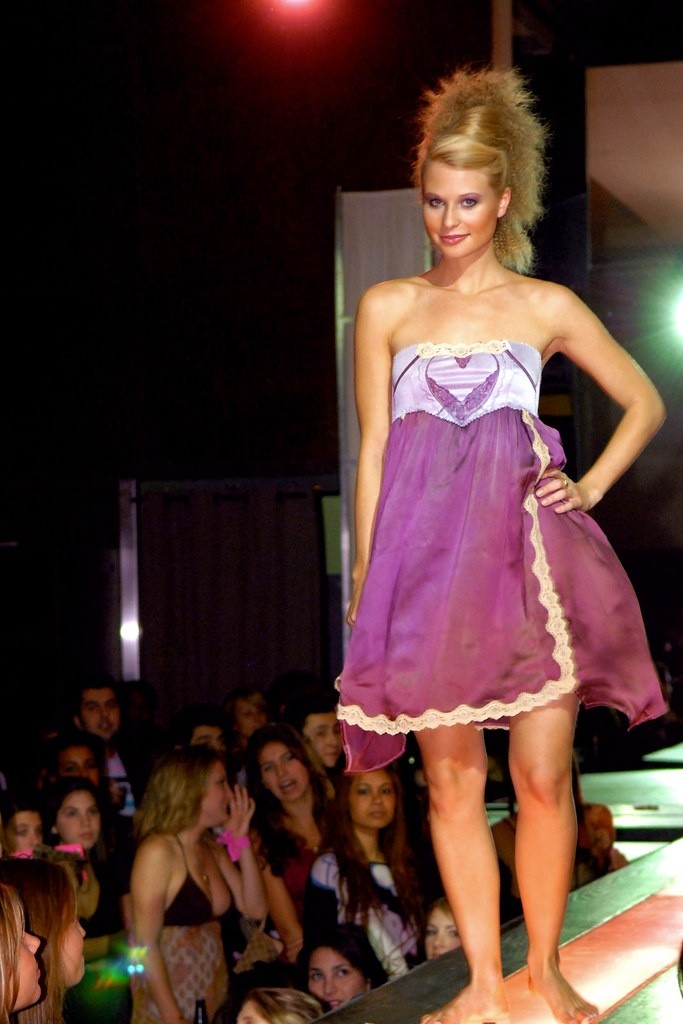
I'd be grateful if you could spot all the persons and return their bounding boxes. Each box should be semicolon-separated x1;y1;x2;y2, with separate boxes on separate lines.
0;672;630;1024
334;65;668;1024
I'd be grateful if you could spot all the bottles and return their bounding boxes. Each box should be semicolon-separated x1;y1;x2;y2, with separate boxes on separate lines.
192;999;209;1024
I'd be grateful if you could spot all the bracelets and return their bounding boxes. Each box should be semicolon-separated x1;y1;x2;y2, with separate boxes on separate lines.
217;829;251;861
